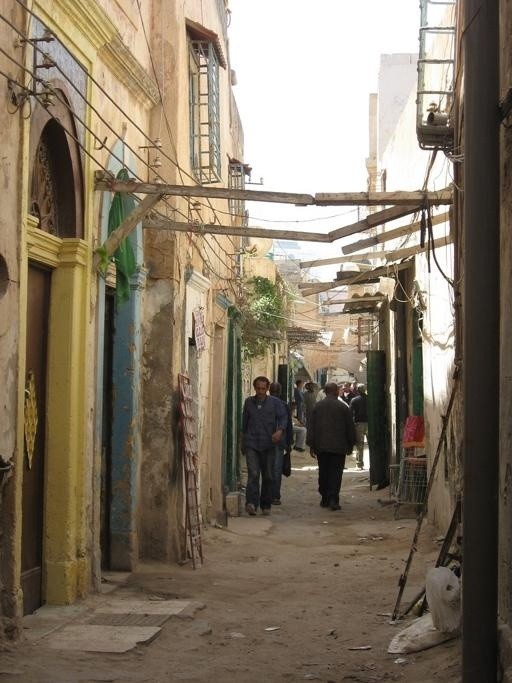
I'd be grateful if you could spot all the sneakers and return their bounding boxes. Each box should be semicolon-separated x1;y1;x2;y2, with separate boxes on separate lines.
272;499;281;505
245;502;256;516
263;508;271;515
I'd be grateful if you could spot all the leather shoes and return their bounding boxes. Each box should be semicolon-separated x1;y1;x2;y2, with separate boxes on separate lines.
329;499;341;510
320;500;329;507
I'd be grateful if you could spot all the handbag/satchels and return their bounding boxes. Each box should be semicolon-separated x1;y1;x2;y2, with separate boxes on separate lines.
283;452;291;477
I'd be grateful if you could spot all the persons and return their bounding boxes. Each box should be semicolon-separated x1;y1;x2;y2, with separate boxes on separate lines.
241;377;288;515
306;382;356;511
350;384;369;470
292;379;357;451
269;383;293;504
